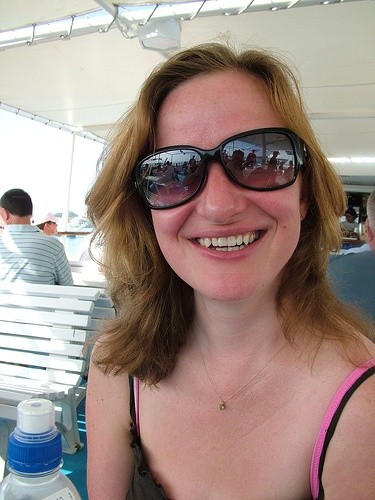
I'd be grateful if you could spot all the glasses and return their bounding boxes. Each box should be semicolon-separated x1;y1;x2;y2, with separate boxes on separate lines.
134;127;309;210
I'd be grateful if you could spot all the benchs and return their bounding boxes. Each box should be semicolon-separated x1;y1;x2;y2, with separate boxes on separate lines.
0;280;119;454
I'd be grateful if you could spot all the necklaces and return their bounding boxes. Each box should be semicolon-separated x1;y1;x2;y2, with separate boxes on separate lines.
186;325;294;411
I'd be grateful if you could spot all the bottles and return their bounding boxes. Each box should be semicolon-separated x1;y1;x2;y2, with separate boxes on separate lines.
1;399;83;500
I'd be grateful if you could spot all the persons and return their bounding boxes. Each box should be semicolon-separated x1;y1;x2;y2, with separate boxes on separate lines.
79;44;374;500
0;188;74;286
326;195;374;317
340;206;360;236
34;212;59;236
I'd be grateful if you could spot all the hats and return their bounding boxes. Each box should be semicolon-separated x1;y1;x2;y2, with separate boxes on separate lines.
30;211;57;226
